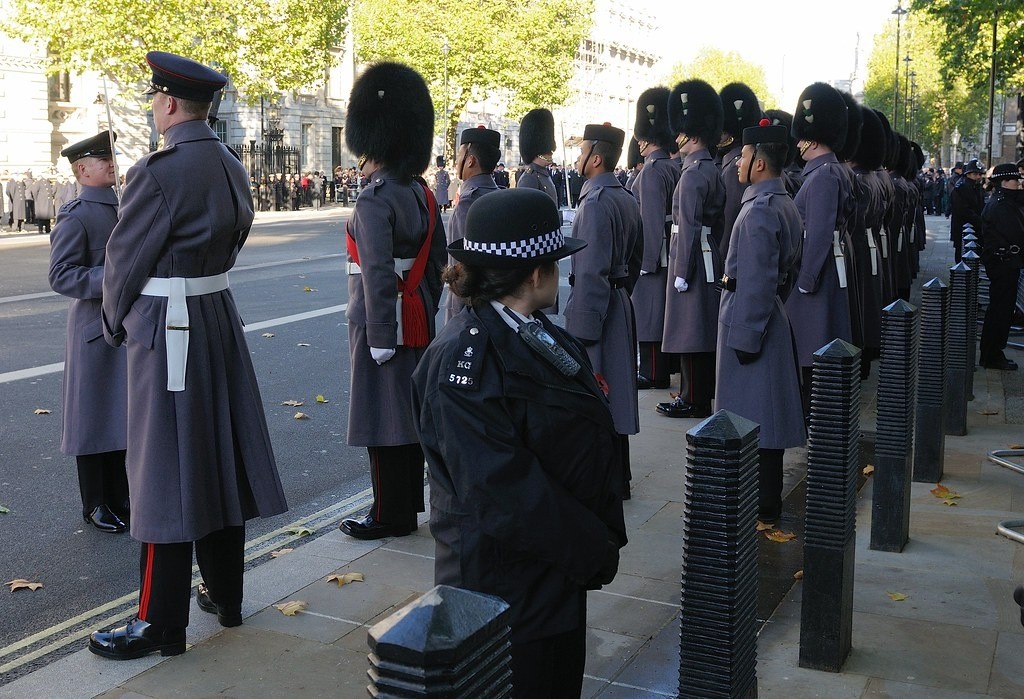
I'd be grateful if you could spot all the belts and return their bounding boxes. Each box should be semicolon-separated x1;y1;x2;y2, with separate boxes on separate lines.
671;224;715;287
720;273;784;295
801;223;916;288
140;270;231;391
346;257;417;347
567;273;626;289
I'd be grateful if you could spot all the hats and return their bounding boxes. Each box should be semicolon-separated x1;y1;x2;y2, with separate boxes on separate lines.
460;126;501;147
437;155;446;168
628;80;925;181
141;51;227;102
583;122;626;146
551;163;557;167
445;187;588;271
520;109;556;165
988;164;1024;182
342;62;435;178
963;160;987;175
61;130;117;164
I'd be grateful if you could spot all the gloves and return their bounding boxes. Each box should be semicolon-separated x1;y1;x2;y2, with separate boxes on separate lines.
735;350;761;365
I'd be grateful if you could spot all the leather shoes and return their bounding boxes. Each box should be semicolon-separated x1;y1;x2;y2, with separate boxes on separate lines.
340;512;419;540
622;481;632;500
83;504;127;533
197;583;243;626
656;394;712;417
670;368;681;374
89;618;187;661
636;376;669;389
979;356;1018;370
114;501;130;518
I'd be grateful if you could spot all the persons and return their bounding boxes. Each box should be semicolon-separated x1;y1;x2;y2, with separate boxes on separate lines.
407;188;633;699
434;77;1024;529
88;50;290;660
247;165;371;212
337;118;449;540
0;168;128;235
45;131;129;535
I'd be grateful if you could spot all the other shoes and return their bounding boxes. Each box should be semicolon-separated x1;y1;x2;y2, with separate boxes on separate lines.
759;512;778;523
